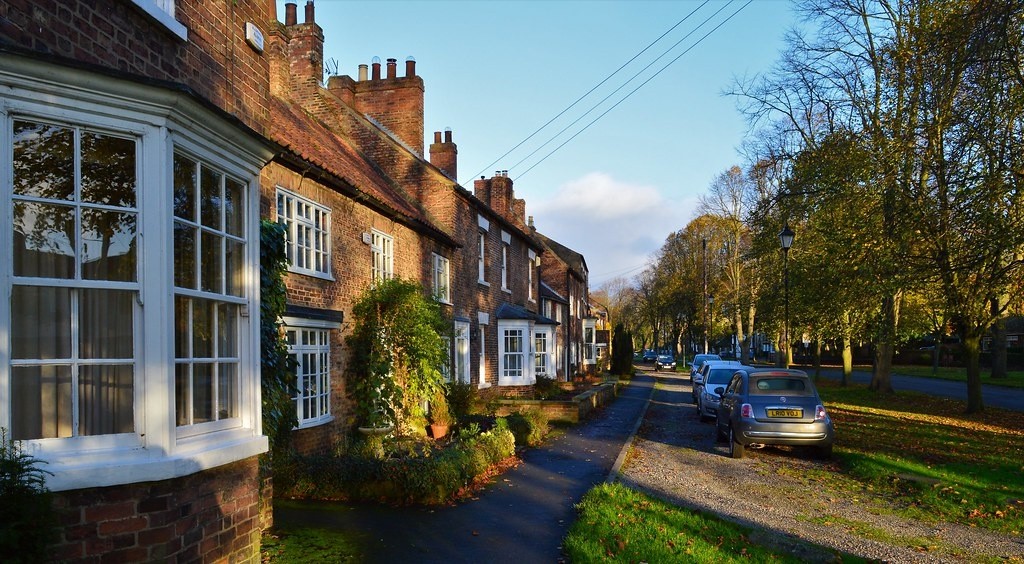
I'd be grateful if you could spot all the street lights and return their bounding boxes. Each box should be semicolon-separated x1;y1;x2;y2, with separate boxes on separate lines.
777;220;797;372
708;292;716;356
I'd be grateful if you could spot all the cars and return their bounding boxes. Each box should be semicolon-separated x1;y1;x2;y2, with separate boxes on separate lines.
714;368;834;463
695;365;756;423
655;355;677;373
688;354;721;383
642;352;658;364
718;352;736;360
692;360;743;402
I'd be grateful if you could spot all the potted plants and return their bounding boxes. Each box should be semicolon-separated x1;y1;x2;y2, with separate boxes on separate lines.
573;372;584;383
431;391;452;439
